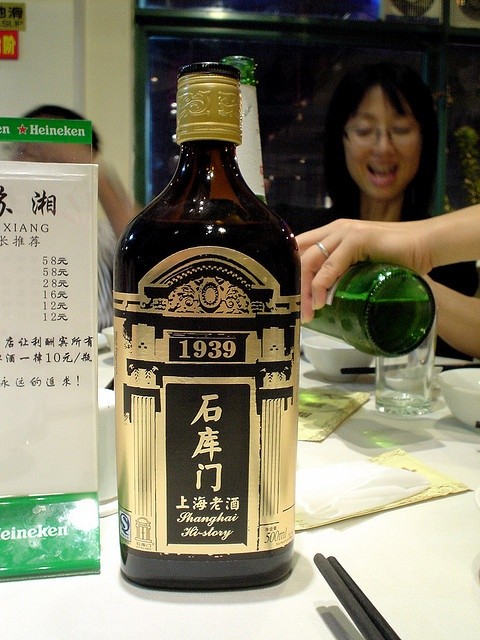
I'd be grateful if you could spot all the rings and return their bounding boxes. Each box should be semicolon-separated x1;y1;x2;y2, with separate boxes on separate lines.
315;241;331;258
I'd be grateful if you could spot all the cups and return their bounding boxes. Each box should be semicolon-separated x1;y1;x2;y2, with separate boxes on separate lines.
374;315;437;417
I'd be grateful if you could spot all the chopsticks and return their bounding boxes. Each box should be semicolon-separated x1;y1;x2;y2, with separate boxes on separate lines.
313;550;401;640
340;361;480;375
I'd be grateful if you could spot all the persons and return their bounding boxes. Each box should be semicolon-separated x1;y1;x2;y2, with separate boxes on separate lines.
14;106;142;331
293;202;480;359
287;54;480;360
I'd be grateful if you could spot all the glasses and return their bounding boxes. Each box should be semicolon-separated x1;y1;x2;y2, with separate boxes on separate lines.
342;122;420;146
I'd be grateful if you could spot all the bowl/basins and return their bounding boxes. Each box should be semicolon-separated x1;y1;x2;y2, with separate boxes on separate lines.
297;334;374;383
437;367;480;427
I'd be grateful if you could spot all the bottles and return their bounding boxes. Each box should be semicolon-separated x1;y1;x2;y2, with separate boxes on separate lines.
303;261;436;357
219;51;272;213
109;62;303;593
